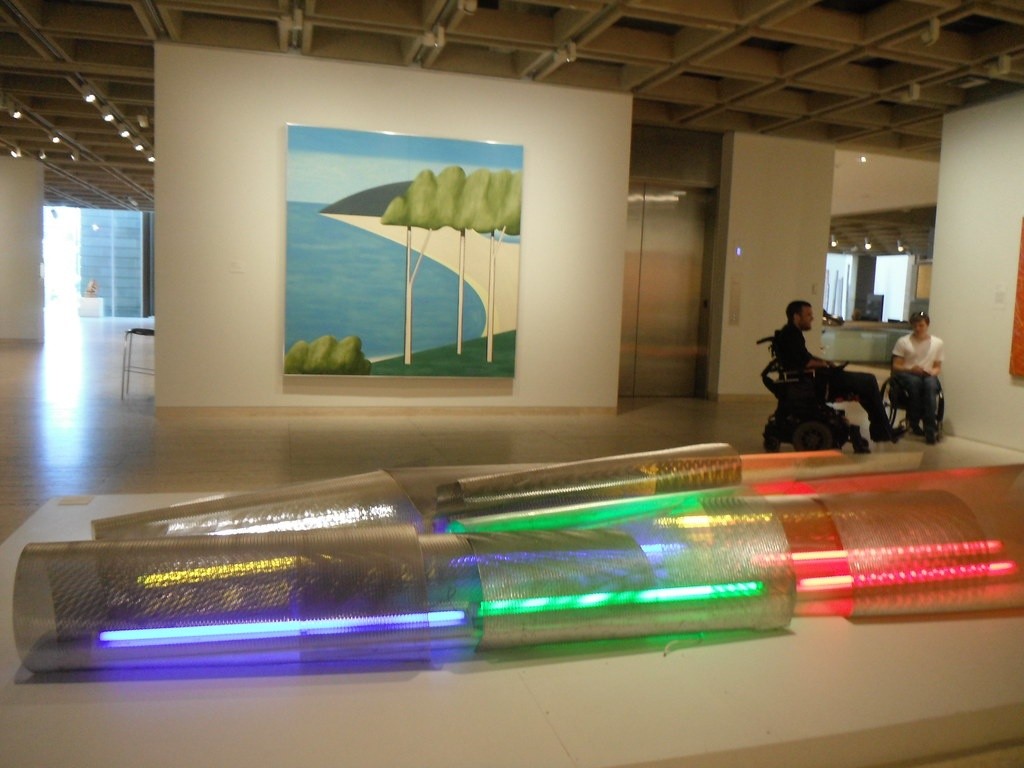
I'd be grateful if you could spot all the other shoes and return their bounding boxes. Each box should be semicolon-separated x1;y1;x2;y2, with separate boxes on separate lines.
910;421;923;435
871;425;905;442
925;432;936;444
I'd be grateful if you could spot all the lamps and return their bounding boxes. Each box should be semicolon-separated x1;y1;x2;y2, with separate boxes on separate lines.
39;151;47;160
81;85;96;103
134;140;143;151
8;104;22;119
864;237;871;250
101;107;114;122
49;132;61;144
147;151;155;164
71;149;79;162
831;234;836;246
118;123;130;138
897;240;904;253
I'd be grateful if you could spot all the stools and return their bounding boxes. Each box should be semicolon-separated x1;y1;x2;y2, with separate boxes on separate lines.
121;329;154;399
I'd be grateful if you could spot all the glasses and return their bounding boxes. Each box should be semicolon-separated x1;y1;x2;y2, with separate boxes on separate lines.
912;311;926;320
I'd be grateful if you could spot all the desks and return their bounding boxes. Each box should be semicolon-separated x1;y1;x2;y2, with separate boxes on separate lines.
822;320;914;364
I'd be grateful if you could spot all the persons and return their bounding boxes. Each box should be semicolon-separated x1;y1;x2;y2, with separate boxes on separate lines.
776;301;903;442
892;313;944;446
